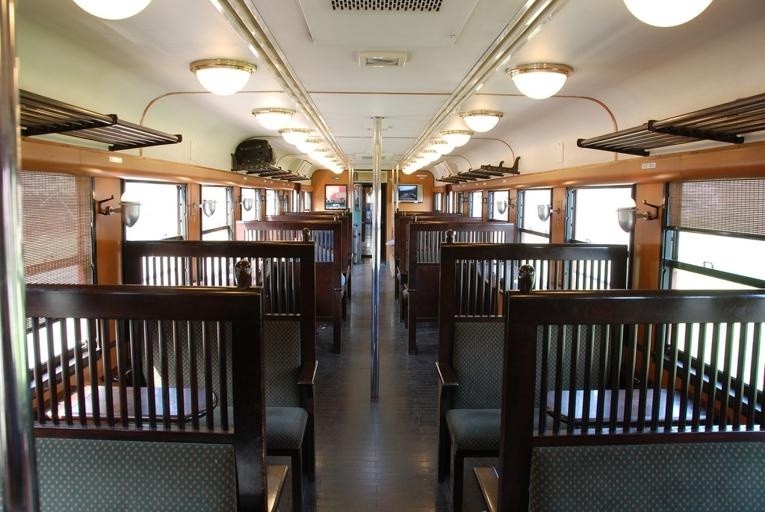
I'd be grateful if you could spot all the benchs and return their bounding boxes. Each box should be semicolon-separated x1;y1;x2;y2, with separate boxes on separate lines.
23;204;353;512
394;205;765;512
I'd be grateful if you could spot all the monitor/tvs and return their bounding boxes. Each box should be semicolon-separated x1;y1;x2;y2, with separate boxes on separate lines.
394;184;423;203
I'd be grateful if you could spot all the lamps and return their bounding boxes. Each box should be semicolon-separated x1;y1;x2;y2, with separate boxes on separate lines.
251;108;320;153
505;63;575;100
429;107;505;156
189;58;258;95
